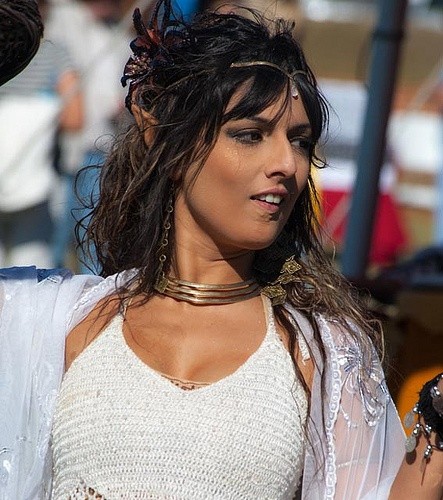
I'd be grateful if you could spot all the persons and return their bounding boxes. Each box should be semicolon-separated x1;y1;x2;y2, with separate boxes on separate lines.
0;0;306;284
0;0;443;500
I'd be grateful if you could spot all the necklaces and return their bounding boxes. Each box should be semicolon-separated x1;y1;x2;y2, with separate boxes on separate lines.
155;272;260;303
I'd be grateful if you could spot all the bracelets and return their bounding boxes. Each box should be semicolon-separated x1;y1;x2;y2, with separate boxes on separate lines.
412;373;443;458
